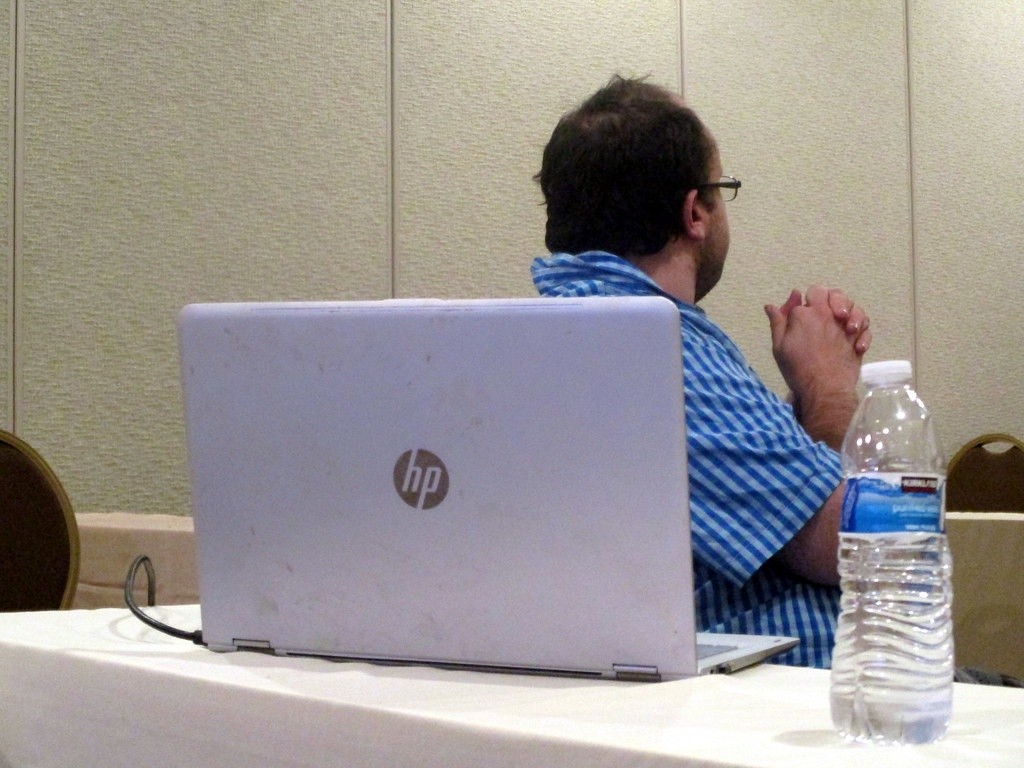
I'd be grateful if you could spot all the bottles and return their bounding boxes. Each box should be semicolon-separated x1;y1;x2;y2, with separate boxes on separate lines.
832;360;955;749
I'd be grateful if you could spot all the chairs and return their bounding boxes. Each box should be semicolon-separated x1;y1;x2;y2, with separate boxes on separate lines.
946;433;1024;513
0;426;80;613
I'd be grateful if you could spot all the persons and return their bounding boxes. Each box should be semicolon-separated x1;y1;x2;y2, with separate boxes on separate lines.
531;71;1024;688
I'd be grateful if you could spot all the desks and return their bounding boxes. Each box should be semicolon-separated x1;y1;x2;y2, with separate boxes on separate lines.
0;604;1024;768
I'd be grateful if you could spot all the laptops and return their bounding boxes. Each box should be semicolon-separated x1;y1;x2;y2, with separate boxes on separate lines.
177;295;801;683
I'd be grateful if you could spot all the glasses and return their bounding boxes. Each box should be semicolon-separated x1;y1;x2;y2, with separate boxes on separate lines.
688;176;742;201
